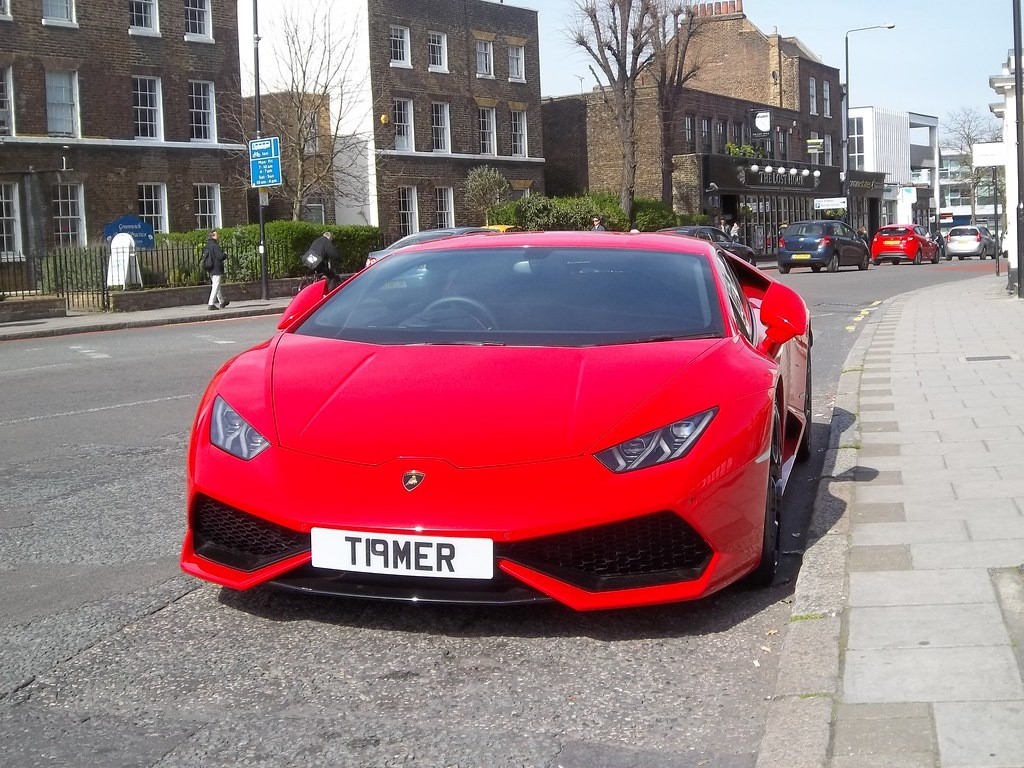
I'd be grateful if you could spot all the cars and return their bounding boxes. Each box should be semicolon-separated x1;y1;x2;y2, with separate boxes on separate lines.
779;220;870;272
651;223;757;269
943;226;995;261
481;226;529;235
365;225;500;308
763;222;782;246
1002;230;1009;258
871;224;940;265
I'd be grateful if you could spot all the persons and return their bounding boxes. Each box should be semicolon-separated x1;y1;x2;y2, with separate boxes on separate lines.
717;216;741;244
302;231;340;294
630;223;640;233
857;225;868;246
591;214;605;231
203;230;230;311
932;227;944;264
779;220;789;237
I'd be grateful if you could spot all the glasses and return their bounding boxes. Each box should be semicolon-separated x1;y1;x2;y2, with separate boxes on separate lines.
593;220;599;222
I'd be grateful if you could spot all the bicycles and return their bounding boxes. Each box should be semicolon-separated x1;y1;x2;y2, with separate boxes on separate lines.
296;259;346;302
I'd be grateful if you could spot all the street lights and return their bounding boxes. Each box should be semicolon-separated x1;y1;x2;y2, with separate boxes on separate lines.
978;141;1000;277
844;24;896;224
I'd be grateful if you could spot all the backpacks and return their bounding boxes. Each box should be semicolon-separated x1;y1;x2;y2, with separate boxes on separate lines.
202;250;214;270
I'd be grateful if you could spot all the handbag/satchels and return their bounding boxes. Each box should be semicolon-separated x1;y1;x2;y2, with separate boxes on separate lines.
302;249;323;269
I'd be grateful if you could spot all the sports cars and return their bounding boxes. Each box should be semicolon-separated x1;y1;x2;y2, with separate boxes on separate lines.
179;231;811;609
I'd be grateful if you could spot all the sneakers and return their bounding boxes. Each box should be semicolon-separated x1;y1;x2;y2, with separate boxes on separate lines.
220;302;229;308
208;305;219;310
